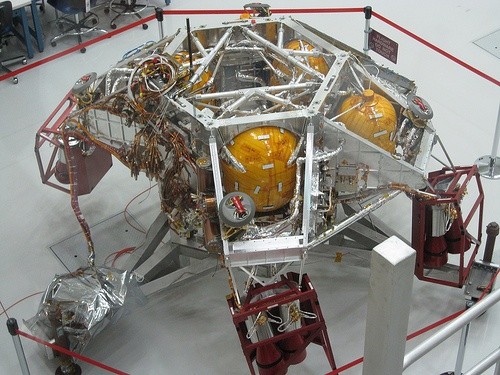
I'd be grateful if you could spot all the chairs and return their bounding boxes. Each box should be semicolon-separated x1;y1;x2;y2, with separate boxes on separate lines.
0;1;27;84
47;0;159;53
